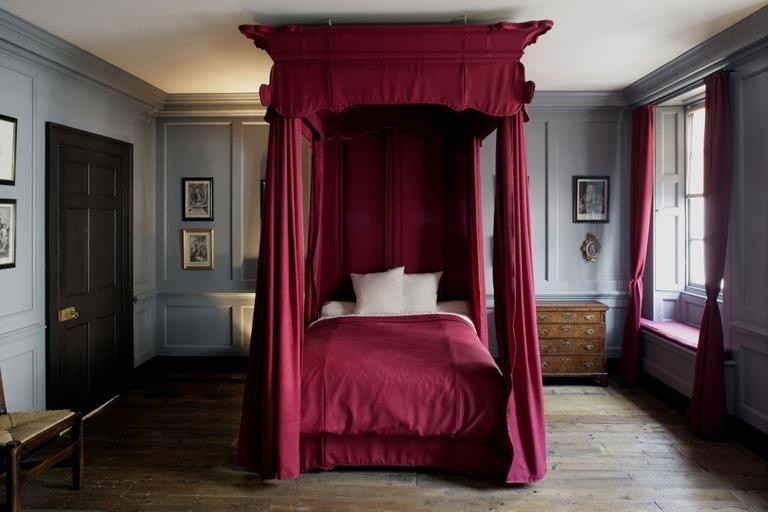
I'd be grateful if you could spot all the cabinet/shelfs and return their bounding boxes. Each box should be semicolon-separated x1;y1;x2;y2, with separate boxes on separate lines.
534;300;608;380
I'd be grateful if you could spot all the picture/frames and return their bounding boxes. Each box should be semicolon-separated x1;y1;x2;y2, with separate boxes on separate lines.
182;226;216;271
572;174;611;224
181;175;215;222
0;198;17;269
579;231;602;264
0;113;19;189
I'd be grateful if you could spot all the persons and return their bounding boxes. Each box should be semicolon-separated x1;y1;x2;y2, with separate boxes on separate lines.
579;184;604;215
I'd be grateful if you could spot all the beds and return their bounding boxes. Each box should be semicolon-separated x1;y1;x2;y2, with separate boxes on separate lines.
236;16;554;490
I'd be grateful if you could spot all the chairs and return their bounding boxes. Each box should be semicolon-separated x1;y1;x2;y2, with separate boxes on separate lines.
0;368;84;512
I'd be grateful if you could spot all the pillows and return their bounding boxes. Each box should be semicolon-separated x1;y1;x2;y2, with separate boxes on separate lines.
387;268;444;315
347;266;407;315
